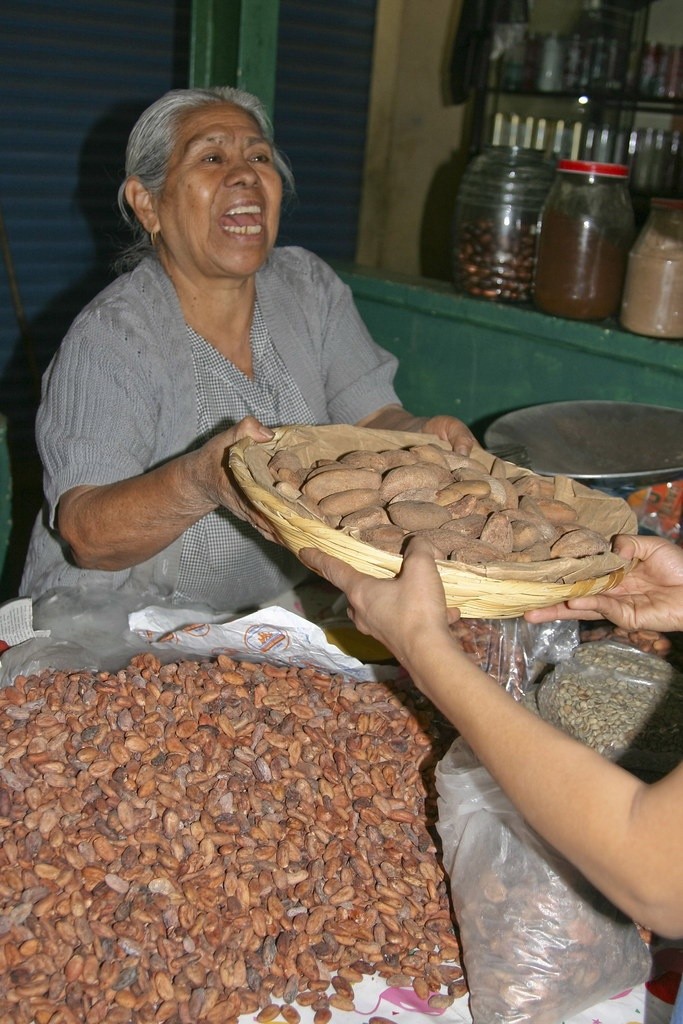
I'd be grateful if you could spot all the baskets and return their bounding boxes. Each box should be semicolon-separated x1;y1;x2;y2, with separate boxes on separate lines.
229;424;640;620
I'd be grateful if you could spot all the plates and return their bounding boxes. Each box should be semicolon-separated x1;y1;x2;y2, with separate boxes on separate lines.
479;400;683;490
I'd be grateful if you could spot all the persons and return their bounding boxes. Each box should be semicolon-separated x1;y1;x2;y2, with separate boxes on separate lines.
299;534;683;1024
18;87;481;612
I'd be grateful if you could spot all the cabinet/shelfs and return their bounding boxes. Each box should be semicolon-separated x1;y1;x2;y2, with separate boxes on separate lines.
475;1;682;228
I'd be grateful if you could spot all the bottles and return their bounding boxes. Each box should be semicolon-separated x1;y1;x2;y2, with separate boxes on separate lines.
504;24;683;99
489;111;683;197
449;144;683;339
